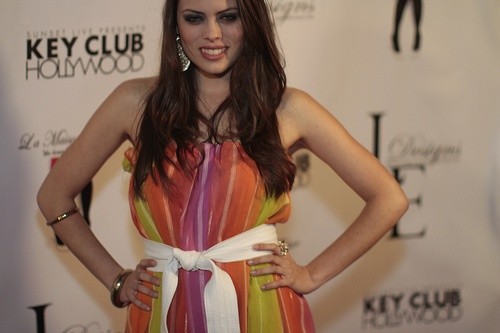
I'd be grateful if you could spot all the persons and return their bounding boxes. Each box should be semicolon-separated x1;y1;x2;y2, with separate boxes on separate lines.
37;0;410;333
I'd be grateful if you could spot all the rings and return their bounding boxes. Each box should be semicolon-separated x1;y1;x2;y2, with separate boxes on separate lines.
278;240;288;256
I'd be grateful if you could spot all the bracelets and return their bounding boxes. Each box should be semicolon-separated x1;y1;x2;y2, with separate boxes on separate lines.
45;203;80;227
110;269;135;308
